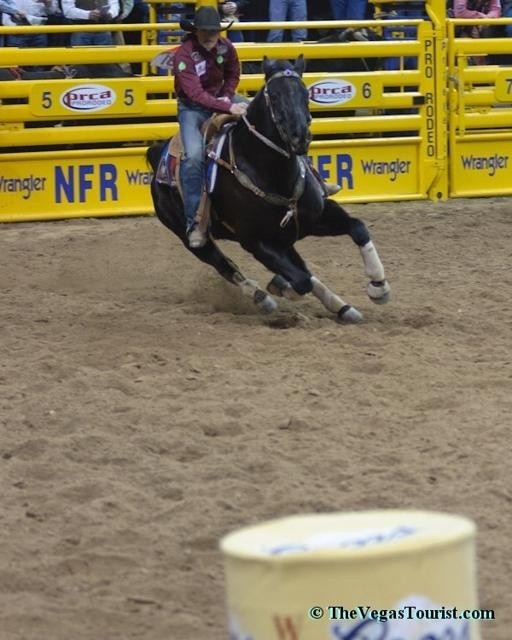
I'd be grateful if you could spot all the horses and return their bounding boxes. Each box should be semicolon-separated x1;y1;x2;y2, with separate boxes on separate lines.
144;53;392;324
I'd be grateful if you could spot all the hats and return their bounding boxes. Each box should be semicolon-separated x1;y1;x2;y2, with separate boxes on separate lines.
180;5;234;32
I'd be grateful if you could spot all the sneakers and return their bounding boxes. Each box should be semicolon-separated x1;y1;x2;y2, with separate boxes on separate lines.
320;180;341;197
188;223;208;248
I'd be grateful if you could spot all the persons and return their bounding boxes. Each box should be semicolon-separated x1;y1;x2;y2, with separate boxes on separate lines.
1;1;511;156
172;6;343;249
